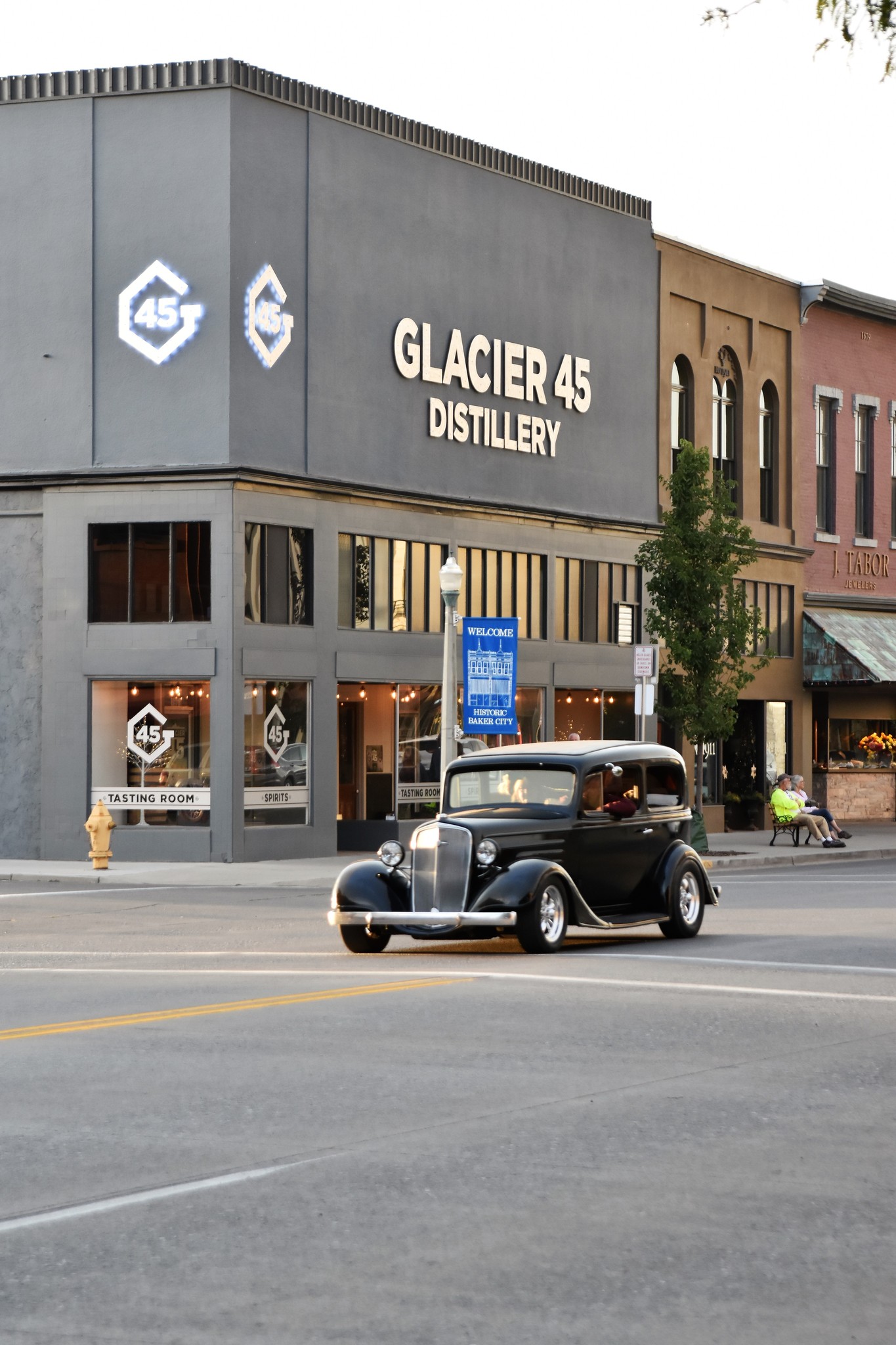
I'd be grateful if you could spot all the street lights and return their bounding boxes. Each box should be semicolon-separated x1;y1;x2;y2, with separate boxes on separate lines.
438;557;464;812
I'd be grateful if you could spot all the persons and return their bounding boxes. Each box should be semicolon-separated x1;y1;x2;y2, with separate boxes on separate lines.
399;739;441;819
568;733;580;740
496;774;636;818
790;775;853;847
770;773;844;848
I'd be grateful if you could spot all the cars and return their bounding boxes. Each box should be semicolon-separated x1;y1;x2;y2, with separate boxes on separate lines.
159;741;306;825
326;739;720;954
397;734;489;771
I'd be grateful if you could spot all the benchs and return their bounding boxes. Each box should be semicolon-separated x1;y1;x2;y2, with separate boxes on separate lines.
767;801;811;847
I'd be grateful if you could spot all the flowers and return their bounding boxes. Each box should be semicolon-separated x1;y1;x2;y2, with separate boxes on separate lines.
857;733;896;760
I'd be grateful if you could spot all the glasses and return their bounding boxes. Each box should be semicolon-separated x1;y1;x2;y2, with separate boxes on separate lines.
800;781;804;783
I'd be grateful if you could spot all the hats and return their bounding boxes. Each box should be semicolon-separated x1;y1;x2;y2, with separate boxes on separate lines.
778;774;793;781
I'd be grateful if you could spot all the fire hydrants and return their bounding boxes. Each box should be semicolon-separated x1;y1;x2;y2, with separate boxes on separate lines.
83;797;117;870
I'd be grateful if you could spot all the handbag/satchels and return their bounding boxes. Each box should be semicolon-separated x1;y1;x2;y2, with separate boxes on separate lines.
804;797;818;808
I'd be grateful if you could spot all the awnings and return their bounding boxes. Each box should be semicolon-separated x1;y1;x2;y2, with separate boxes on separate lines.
802;591;896;687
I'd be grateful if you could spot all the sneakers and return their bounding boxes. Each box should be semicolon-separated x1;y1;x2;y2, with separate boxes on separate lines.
823;839;835;848
837;830;852;839
828;838;846;847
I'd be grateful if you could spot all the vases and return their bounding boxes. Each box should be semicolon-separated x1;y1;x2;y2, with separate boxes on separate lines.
878;747;892;768
